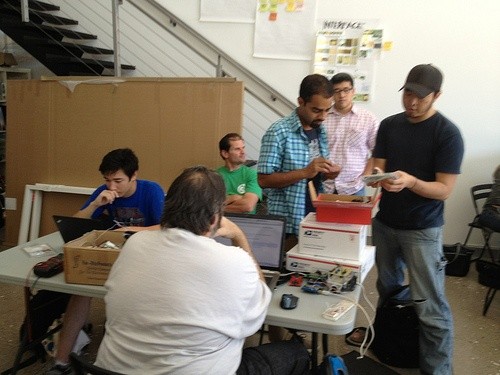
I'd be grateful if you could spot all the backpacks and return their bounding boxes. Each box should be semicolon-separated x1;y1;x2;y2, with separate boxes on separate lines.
18;289;73;362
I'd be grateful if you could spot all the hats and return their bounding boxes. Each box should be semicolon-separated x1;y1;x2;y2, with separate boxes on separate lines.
398;64;443;98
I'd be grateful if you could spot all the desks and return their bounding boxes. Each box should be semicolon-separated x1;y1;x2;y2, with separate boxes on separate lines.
0;232;362;375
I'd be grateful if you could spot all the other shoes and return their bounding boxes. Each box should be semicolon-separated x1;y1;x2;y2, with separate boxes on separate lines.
345;328;370;348
282;332;319;350
44;364;78;375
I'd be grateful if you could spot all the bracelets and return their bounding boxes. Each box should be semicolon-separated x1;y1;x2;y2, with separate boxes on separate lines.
407;177;418;189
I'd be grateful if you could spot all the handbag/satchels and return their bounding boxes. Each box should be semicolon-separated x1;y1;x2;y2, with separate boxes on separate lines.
443;243;475;276
474;260;499;289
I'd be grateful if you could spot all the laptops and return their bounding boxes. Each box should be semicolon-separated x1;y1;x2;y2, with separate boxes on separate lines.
214;212;287;291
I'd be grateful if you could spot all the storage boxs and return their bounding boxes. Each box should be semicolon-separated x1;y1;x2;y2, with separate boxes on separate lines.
0;53;18;67
63;229;125;285
286;192;382;282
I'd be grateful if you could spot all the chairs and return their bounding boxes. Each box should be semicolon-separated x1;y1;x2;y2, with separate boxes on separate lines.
463;182;500;264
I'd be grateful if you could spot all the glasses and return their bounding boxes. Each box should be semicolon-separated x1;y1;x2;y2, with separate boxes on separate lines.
333;86;352;94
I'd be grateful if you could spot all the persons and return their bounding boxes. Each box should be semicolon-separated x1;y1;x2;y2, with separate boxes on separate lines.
359;63;465;375
94;166;310;375
322;72;376;198
213;134;263;216
43;148;168;375
257;73;343;353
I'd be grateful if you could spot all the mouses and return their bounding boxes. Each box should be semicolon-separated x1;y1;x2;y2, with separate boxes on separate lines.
280;294;298;309
